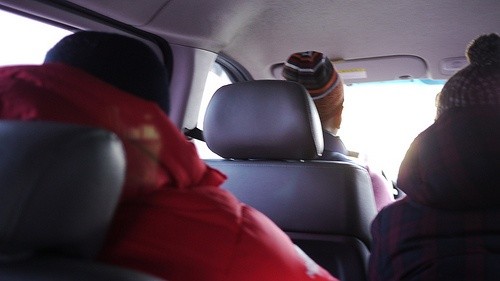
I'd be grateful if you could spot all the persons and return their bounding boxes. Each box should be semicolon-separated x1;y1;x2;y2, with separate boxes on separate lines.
282;51;395;211
368;33;500;281
0;31;338;281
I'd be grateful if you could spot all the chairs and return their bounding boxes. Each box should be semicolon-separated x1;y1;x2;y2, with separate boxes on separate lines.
0;120;163;281
199;79;378;281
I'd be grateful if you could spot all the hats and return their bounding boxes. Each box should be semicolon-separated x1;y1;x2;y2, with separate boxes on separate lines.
435;33;500;120
282;51;344;121
43;29;171;114
